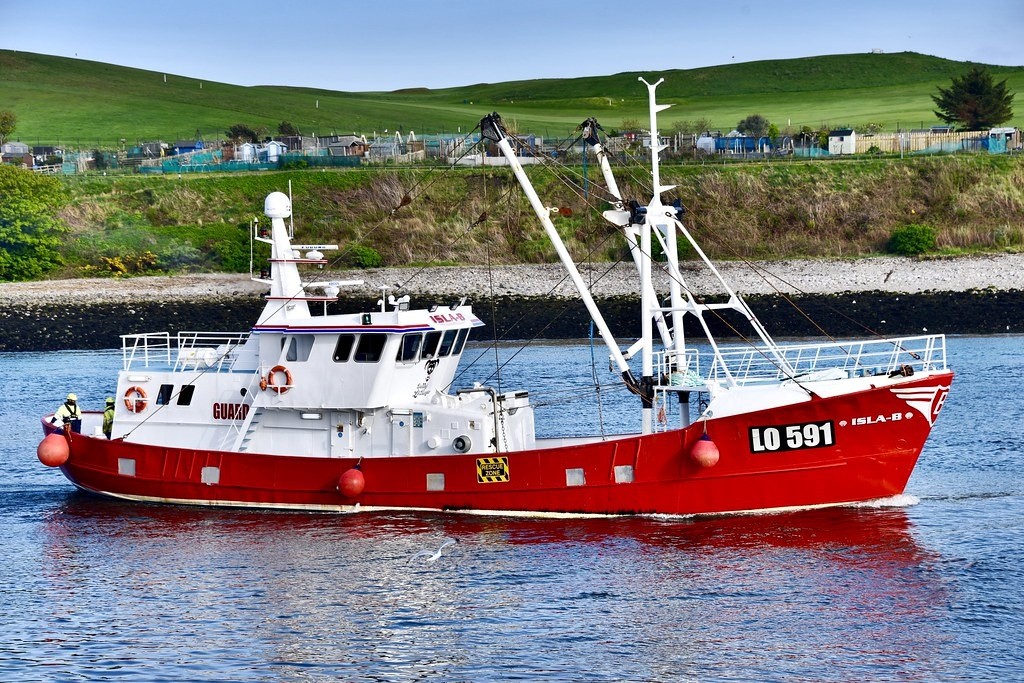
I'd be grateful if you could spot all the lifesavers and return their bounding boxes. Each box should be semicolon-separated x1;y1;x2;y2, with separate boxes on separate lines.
124;387;147;413
268;365;292;393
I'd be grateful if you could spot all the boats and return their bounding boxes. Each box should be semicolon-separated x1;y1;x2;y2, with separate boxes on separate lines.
38;76;956;517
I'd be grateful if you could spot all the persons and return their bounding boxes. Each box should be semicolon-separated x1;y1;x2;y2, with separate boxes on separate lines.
48;393;82;434
102;397;115;441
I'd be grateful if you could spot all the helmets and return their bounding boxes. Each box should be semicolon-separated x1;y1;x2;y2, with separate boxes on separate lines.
67;393;77;400
106;397;115;403
